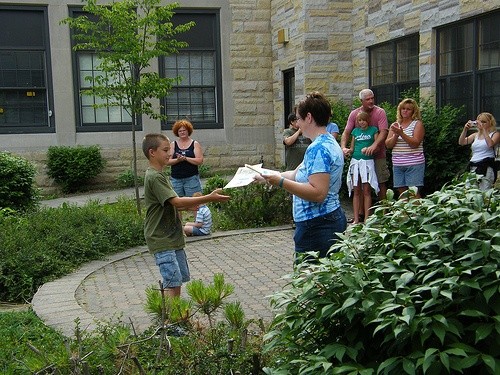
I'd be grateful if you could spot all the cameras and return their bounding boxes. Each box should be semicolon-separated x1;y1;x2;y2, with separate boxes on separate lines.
469;121;478;128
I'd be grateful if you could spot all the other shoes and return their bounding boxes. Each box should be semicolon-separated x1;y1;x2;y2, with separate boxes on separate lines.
155;323;188;336
347;214;365;223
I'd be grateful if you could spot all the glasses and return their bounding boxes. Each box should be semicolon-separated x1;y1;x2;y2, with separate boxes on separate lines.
293;116;302;123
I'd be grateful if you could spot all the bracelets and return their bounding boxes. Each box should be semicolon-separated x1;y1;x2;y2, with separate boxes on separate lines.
279;178;284;188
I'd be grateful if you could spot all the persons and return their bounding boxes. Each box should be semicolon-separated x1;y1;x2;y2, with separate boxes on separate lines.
168;120;203;223
459;112;500;186
184;193;213;237
282;89;426;228
252;91;345;265
142;133;231;334
